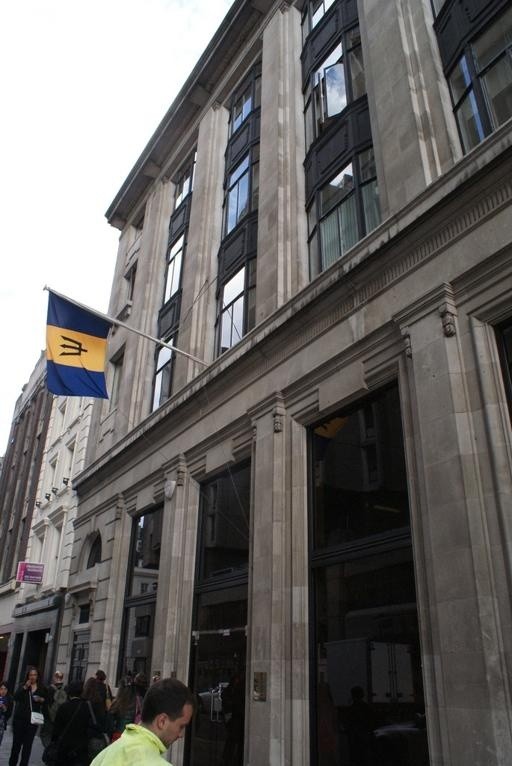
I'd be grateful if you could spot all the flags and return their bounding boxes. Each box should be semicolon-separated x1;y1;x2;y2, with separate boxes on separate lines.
46;290;112;399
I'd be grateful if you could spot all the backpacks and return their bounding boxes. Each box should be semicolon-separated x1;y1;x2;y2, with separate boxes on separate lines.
48;684;68;722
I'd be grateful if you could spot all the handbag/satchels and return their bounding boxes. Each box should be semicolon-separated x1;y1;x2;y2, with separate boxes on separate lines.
105;684;112;712
42;744;58;766
31;712;44;726
134;696;143;725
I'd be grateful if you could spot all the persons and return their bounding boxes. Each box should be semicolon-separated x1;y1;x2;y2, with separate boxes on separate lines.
1;665;195;764
219;669;429;766
